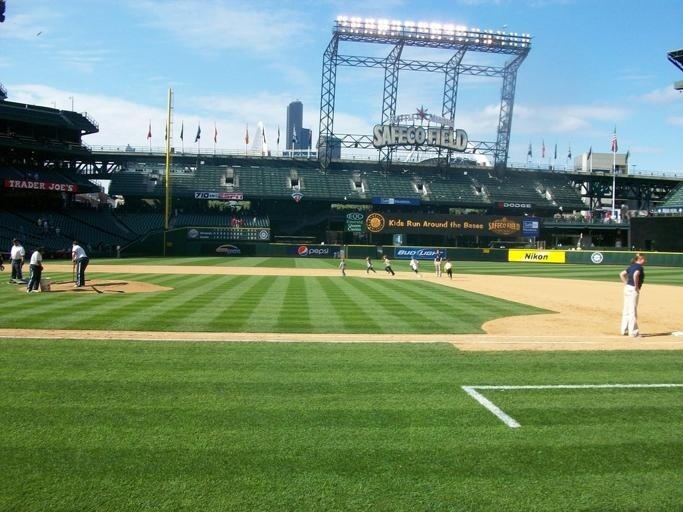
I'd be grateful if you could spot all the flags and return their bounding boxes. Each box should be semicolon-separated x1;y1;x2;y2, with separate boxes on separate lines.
145;119;152;140
527;140;533;159
243;123;250;145
609;123;620;153
261;126;267;145
275;124;280;145
567;144;573;160
553;143;558;160
164;120;167;141
586;146;592;160
194;120;201;143
624;150;629;164
291;123;298;145
213;121;218;143
179;120;183;142
307;134;311;149
541;138;546;157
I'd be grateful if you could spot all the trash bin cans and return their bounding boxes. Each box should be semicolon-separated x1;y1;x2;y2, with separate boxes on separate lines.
111;245;121;258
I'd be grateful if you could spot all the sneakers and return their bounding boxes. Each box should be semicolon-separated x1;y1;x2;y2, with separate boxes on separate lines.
32;290;40;293
73;283;85;288
26;289;32;293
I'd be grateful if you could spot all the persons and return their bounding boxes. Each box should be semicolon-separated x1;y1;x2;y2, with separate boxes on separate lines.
337;258;346;277
6;236;25;284
381;255;395;276
364;256;375;274
37;218;61;236
70;240;89;288
433;256;443;278
618;251;645;338
0;252;5;273
408;255;420;275
231;215;257;228
443;258;453;279
25;246;46;293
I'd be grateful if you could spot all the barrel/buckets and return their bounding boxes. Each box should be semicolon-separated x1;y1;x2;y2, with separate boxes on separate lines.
40;277;51;291
